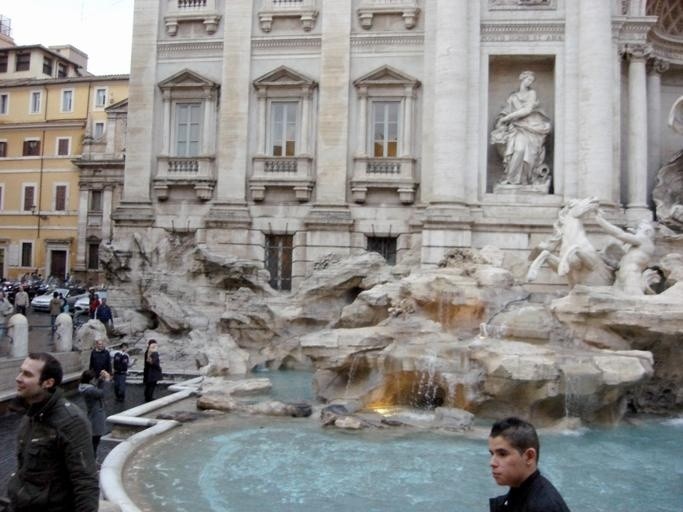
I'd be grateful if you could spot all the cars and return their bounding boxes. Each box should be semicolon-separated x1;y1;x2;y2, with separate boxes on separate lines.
1;274;108;315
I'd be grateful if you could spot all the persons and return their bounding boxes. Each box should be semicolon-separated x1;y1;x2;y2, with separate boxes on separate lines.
0;271;112;339
0;353;98;511
105;342;136;402
142;338;163;402
487;416;569;510
88;337;111;385
78;368;113;456
592;209;658;300
